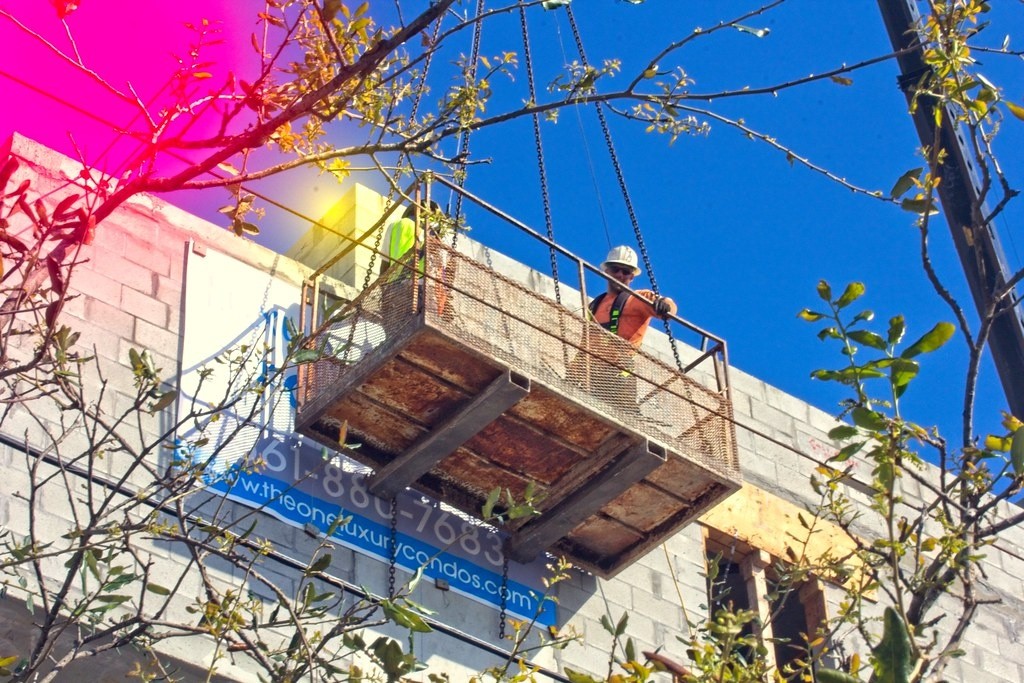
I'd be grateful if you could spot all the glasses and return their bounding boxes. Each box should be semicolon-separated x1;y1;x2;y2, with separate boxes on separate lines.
607;266;633;275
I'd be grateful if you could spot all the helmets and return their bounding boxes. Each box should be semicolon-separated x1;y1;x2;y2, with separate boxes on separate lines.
600;245;642;276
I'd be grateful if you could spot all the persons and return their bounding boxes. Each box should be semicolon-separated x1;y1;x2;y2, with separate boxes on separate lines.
379;199;452;337
568;245;677;414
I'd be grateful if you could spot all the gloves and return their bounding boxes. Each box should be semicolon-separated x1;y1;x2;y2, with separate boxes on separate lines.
652;300;671;315
